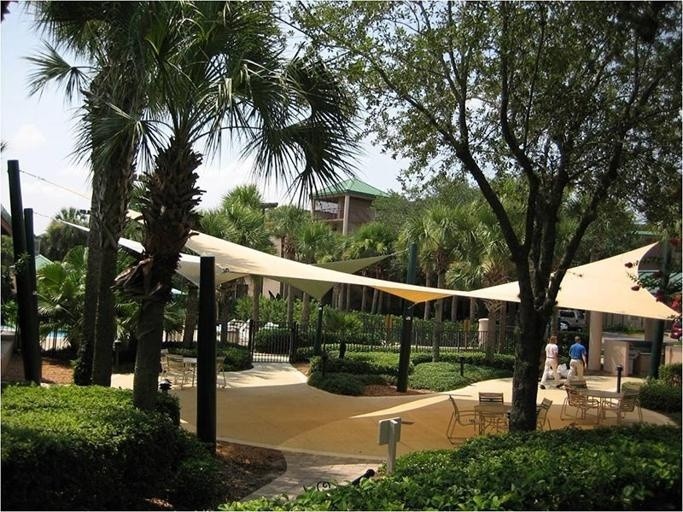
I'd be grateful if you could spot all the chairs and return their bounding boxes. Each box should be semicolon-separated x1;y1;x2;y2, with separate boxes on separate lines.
443;379;645;448
156;346;228;392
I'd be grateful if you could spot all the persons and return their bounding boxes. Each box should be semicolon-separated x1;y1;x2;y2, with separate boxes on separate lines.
568;336;587;380
540;336;565;389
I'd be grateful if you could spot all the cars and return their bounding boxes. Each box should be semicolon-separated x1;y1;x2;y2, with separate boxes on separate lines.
671;316;682;341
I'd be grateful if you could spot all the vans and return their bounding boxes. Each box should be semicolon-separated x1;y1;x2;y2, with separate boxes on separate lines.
558;309;587;330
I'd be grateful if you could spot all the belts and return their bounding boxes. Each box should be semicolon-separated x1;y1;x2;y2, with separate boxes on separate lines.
570;357;582;360
547;355;558;360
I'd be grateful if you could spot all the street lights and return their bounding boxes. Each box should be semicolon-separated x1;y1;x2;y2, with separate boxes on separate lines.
256;198;279;295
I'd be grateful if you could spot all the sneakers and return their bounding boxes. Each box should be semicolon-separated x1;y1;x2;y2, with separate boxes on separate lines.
539;382;564;390
